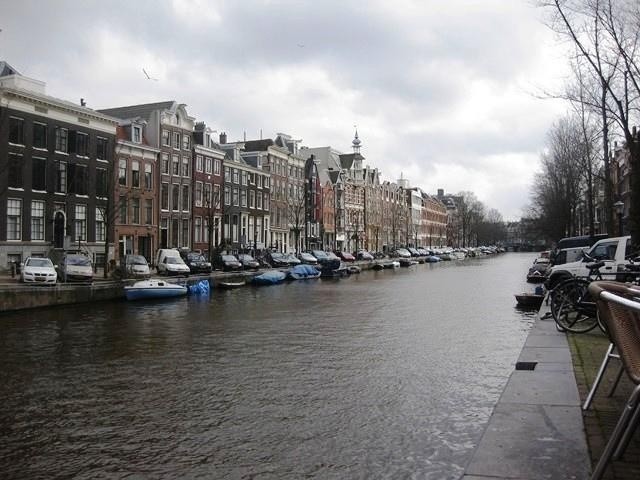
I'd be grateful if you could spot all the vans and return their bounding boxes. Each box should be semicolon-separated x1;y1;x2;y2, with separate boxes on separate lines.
47;248;93;283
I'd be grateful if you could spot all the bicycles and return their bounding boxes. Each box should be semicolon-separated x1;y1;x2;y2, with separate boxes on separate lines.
549;249;640;335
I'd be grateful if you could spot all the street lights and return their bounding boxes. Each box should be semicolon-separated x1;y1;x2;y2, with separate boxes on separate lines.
254;230;258;249
612;198;628;235
593;219;600;234
147;226;152;264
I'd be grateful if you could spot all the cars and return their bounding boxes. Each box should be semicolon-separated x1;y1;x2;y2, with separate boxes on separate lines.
19;255;59;286
115;255;150;278
235;245;506;274
542;229;640;307
209;253;243;273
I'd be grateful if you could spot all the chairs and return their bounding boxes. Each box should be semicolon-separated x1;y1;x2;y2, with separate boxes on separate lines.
581;280;640;479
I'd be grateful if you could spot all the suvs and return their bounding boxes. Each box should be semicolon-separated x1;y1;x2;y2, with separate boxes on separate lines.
179;252;213;274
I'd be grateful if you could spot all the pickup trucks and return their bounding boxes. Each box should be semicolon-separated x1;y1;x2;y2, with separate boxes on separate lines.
154;249;190;276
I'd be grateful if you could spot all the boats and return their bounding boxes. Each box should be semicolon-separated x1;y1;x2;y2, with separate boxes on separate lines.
514;286;546;307
526;248;552;284
125;276;189;301
253;270;287;285
217;275;247;287
285;263;321;280
